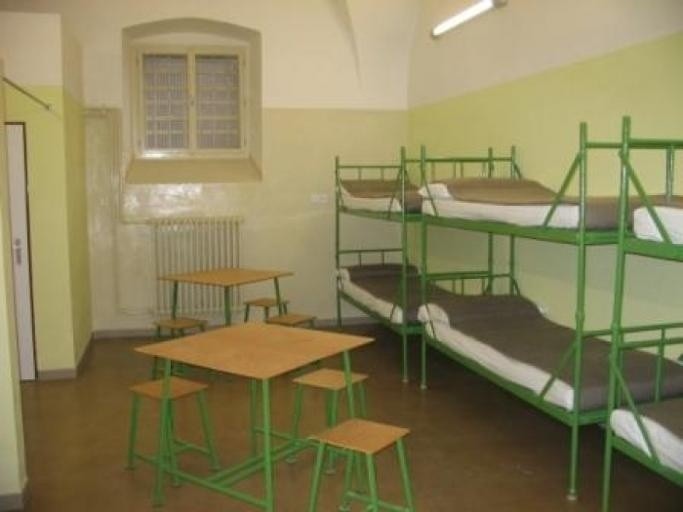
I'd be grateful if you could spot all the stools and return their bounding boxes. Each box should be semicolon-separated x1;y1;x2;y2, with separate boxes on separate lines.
152;317;208;378
309;417;415;511
242;297;290;323
266;312;319;369
130;377;222;489
286;369;370;477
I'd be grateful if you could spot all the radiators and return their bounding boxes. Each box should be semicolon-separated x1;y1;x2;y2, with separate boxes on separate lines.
154;216;246;323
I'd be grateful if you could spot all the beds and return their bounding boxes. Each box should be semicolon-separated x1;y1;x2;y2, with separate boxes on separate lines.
417;122;683;510
332;146;494;384
601;114;682;512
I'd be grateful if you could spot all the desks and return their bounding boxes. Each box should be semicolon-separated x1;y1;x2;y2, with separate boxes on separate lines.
156;267;295;327
134;319;377;512
